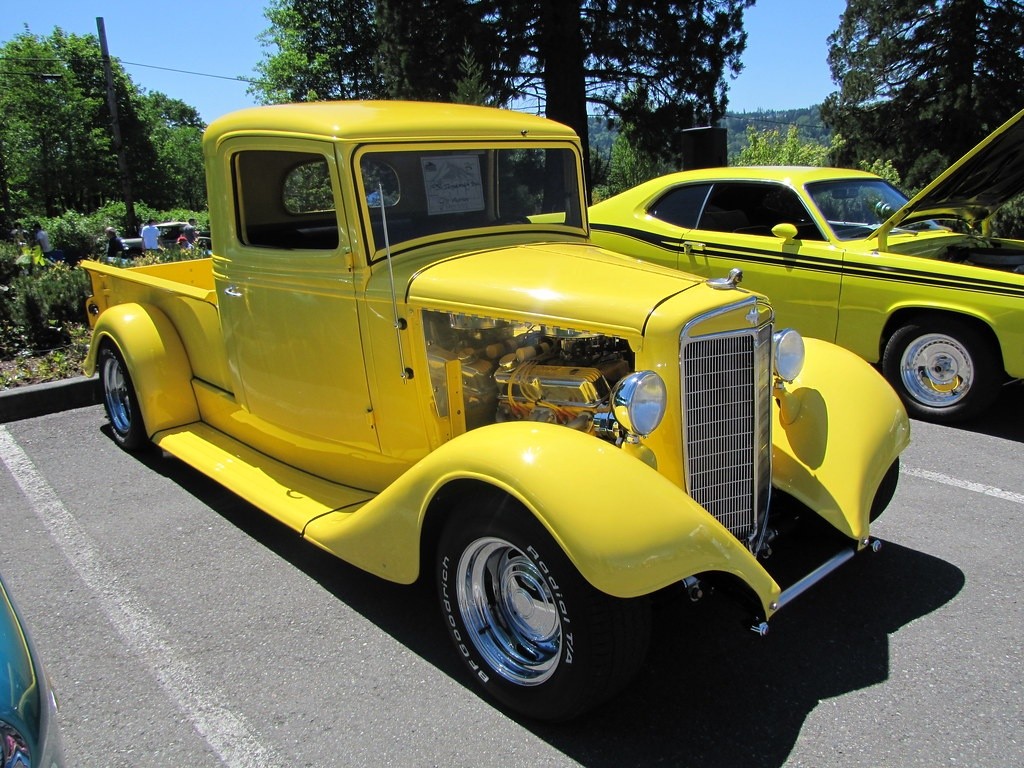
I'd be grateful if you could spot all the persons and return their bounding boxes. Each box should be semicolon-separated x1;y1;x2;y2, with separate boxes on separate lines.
13;220;50;257
177;218;198;248
105;226;126;259
140;219;160;256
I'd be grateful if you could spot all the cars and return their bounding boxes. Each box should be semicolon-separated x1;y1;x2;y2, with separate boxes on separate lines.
121;222;211;259
526;111;1024;428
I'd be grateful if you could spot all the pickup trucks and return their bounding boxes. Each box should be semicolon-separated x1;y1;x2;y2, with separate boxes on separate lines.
81;100;911;725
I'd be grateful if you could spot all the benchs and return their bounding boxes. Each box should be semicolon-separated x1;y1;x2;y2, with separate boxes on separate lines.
245;211;482;249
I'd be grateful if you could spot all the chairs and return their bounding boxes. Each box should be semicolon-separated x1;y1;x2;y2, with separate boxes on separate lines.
699;203;815;236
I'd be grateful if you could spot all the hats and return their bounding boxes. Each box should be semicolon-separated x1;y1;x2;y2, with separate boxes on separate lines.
176;236;188;244
